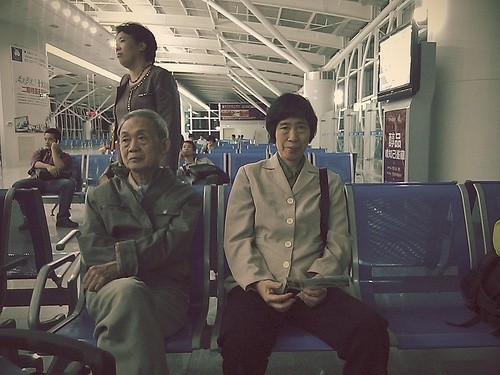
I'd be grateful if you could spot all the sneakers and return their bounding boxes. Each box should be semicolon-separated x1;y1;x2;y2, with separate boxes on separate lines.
18;219;35;230
56;215;79;228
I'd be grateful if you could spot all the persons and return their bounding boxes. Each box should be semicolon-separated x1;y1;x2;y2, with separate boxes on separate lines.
217;93;390;375
76;109;202;375
96;134;244;199
96;21;184;186
12;128;79;231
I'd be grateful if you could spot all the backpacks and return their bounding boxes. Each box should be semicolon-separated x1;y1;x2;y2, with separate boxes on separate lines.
460;249;500;336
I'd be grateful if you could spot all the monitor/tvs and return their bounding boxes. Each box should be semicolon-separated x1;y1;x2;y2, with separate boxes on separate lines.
376;18;419;101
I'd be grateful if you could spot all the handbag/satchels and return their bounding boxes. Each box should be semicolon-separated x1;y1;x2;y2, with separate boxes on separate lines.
188;163;230;183
35;166;72;178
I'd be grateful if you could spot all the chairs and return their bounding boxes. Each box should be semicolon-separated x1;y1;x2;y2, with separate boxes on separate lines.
0;139;500;375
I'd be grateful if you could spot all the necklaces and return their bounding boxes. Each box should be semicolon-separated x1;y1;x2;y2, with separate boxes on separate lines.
129;64;152;83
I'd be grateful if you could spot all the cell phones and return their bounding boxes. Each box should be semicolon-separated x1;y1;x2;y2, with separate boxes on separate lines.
56;138;60;143
